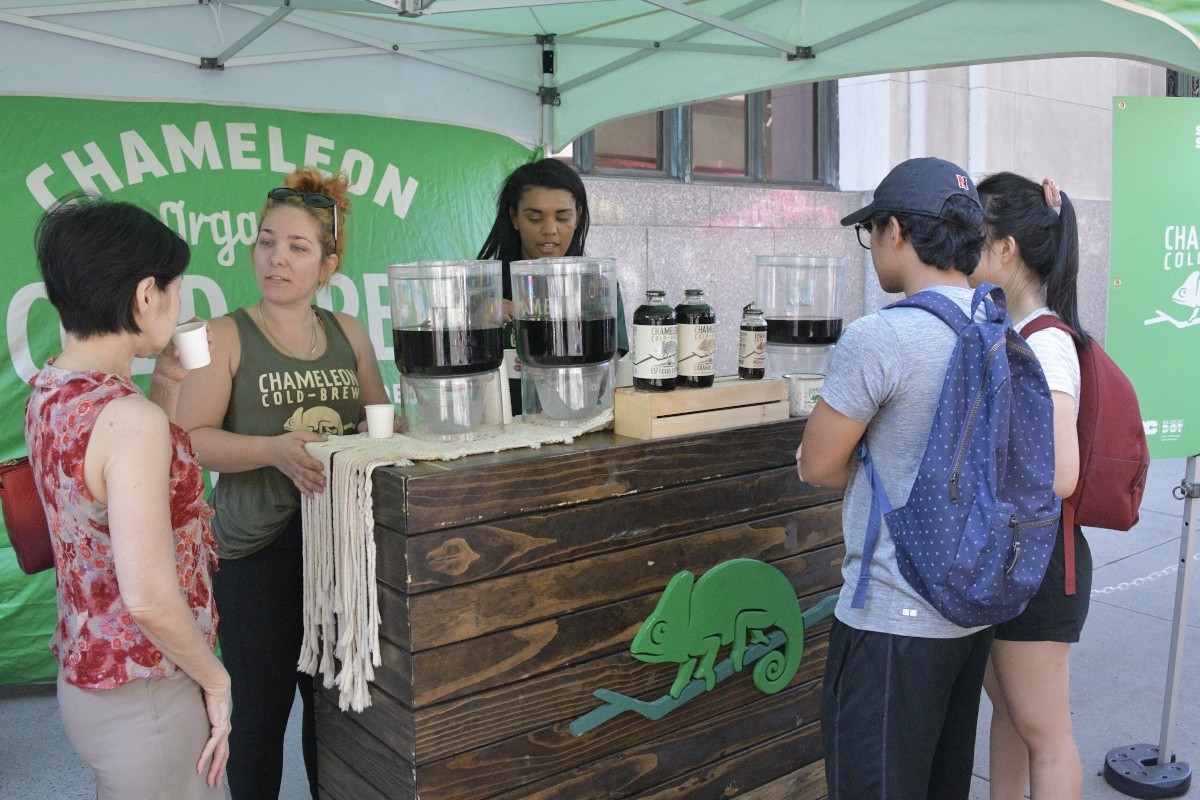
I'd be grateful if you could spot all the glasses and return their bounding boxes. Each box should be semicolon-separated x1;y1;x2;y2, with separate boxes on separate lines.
268;188;338;254
855;217;882;250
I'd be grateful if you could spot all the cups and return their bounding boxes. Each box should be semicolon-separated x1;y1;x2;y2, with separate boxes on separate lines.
503;348;521;378
364;404;394;438
171;322;211;369
783;373;825;417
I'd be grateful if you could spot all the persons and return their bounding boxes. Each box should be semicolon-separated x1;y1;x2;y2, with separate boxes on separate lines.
472;157;629;418
964;172;1092;800
796;157;998;800
175;166;409;800
21;187;235;800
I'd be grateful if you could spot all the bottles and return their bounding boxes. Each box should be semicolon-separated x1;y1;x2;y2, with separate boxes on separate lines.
632;291;678;392
674;288;716;389
738;309;767;379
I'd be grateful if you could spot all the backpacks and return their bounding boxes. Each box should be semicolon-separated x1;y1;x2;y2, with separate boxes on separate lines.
860;284;1061;627
1018;312;1149;534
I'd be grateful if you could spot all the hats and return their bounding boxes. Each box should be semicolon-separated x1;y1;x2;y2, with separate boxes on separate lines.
839;156;984;229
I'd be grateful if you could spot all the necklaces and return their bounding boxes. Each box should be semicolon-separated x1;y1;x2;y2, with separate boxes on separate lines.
257;295;317;358
